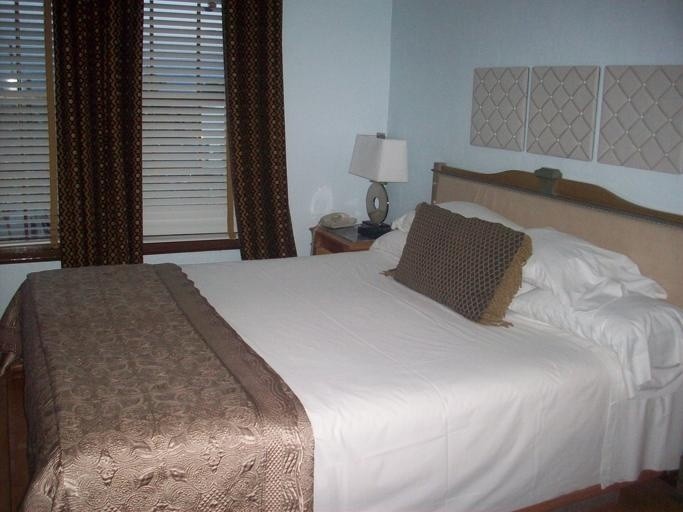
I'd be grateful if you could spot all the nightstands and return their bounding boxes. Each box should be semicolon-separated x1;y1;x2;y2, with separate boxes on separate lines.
309;223;392;256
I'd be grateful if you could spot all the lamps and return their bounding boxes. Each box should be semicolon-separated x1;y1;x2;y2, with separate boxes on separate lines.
348;132;409;239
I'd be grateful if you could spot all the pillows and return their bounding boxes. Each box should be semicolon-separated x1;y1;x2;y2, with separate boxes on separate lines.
508;286;683;390
517;225;668;318
391;200;523;234
380;203;533;328
370;230;536;298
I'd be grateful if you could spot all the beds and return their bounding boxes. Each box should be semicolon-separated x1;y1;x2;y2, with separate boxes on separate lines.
0;161;683;512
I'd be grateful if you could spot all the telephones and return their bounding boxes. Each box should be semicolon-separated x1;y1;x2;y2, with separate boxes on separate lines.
318;212;355;229
